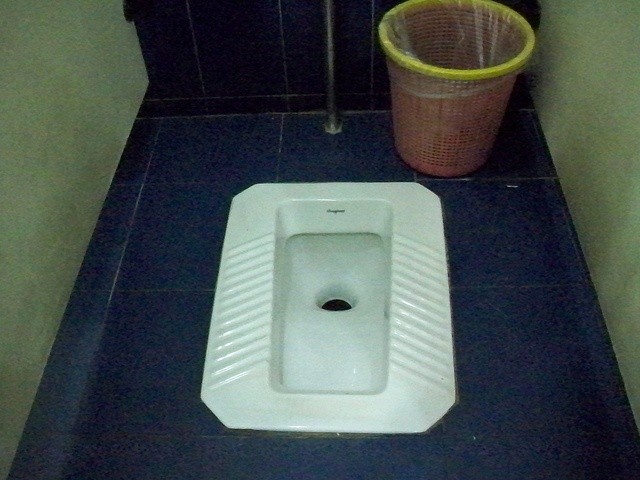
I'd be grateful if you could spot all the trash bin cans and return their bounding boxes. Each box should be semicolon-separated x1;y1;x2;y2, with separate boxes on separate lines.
378;0;536;178
